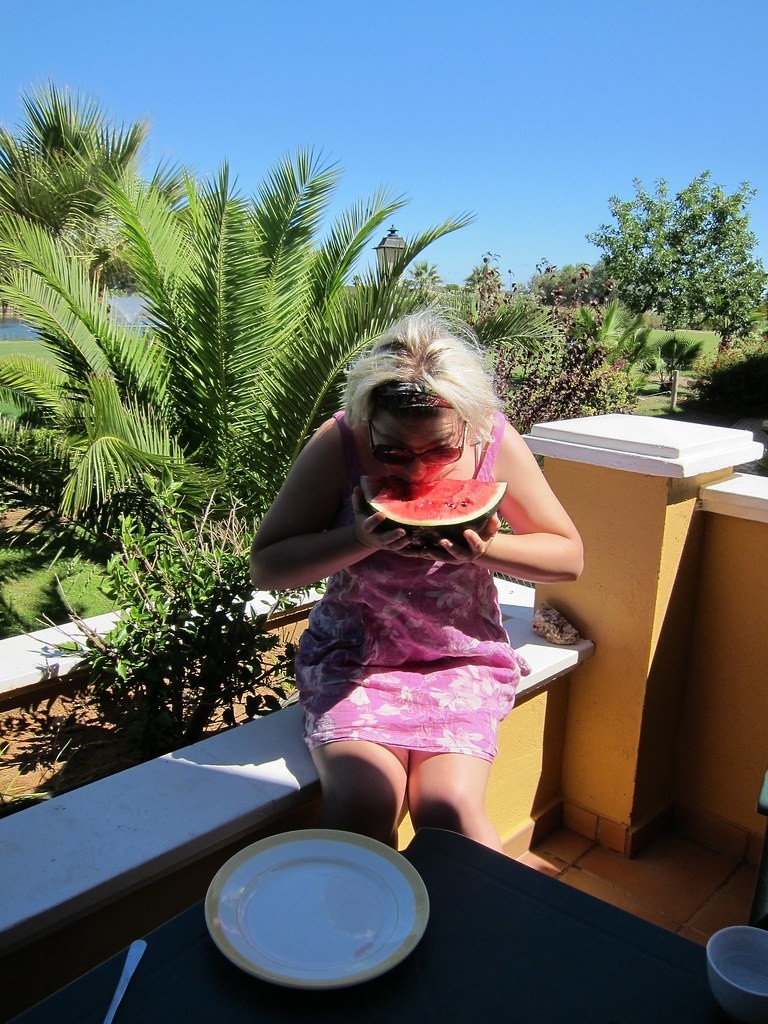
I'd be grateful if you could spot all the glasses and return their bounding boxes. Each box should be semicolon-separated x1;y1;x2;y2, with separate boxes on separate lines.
366;418;467;466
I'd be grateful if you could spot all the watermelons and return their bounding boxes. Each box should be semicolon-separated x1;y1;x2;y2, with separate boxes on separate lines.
359;474;509;544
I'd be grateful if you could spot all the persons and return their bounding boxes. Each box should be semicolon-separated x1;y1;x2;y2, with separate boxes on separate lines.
249;309;586;854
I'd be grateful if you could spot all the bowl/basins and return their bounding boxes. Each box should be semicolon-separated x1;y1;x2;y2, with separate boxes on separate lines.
706;926;768;1017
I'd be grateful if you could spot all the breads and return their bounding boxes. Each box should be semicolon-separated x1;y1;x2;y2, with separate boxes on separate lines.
532;602;579;646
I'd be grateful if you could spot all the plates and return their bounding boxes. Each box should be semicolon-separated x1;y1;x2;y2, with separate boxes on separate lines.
204;828;430;989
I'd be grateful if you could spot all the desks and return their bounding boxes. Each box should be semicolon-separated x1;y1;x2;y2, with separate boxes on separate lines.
6;827;751;1024
